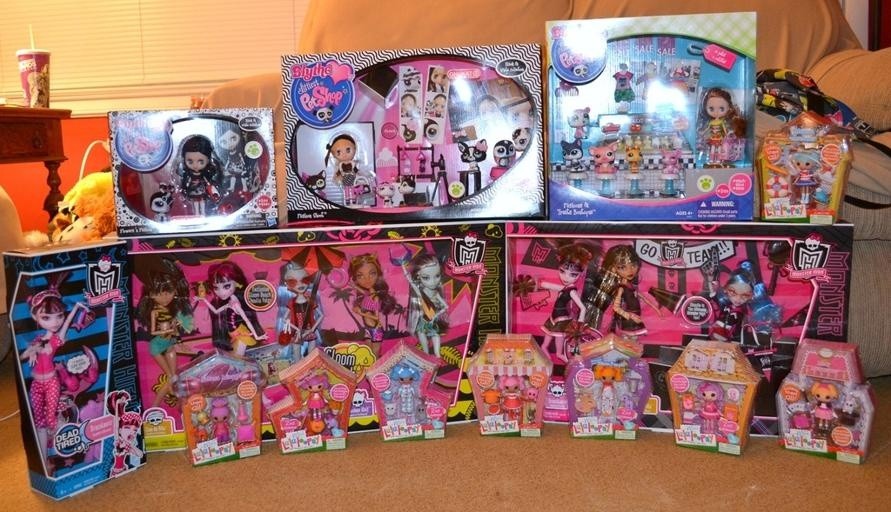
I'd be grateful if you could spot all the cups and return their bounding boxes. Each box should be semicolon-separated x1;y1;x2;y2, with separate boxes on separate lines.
15;49;51;110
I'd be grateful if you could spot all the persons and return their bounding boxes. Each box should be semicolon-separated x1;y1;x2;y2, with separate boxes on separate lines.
19;61;860;476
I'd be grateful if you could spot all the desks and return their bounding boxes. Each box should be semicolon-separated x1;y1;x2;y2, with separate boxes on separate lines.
0;107;72;225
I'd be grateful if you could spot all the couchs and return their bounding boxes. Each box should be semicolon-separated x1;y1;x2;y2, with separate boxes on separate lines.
198;0;891;381
